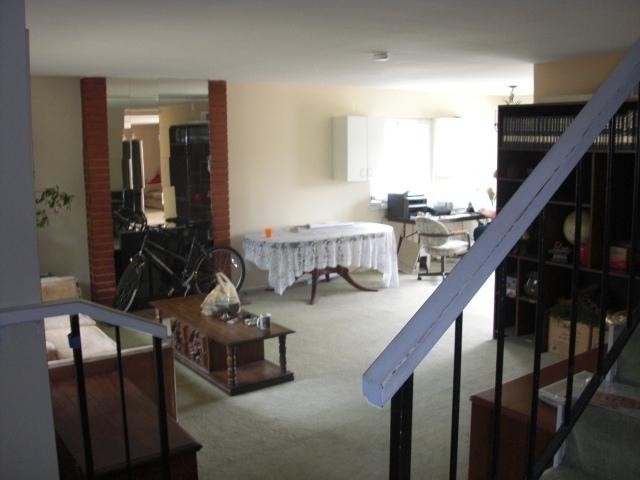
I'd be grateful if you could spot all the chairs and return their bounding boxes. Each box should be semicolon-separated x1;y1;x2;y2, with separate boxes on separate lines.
414;217;470;281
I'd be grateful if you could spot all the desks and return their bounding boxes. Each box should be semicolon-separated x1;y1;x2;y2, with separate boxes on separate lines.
386;211;486;275
244;221;395;306
148;294;295;397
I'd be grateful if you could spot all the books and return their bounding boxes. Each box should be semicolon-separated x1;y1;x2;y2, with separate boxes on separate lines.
501;109;638;150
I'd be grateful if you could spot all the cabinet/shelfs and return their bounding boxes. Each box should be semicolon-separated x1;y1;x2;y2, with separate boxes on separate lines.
493;100;640;345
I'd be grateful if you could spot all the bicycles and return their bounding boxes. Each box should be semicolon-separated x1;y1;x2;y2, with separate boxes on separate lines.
112;210;245;314
117;210;210;229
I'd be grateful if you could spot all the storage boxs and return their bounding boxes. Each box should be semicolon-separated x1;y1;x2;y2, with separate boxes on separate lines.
546;316;609;357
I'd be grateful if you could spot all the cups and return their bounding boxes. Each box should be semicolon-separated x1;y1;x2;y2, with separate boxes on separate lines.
264;226;272;240
256;313;272;331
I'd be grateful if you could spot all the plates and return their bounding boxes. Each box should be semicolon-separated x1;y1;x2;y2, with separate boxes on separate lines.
539;370;595;408
